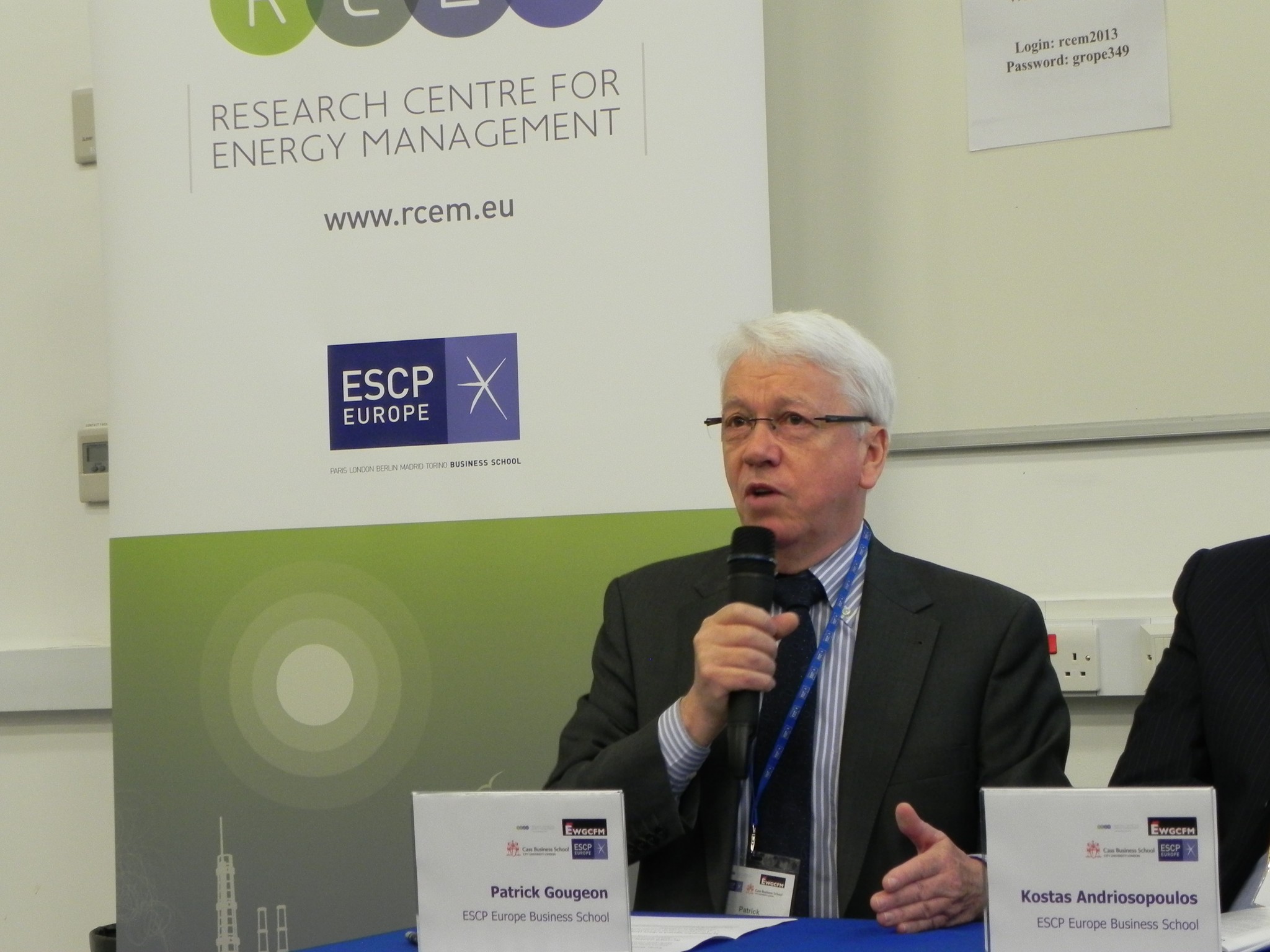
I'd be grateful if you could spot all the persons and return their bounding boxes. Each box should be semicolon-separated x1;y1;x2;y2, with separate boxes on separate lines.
540;308;1070;932
1110;534;1270;909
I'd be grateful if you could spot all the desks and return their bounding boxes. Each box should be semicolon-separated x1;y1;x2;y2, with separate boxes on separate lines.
296;913;1270;952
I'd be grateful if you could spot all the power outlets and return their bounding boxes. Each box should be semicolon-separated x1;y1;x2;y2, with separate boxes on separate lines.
1045;625;1100;693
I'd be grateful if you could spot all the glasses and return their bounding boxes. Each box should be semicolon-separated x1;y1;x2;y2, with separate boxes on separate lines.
703;408;874;446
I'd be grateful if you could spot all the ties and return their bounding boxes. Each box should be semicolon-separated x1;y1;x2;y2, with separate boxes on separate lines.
743;568;830;915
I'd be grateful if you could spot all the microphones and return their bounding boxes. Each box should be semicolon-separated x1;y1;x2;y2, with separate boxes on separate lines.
725;525;777;782
